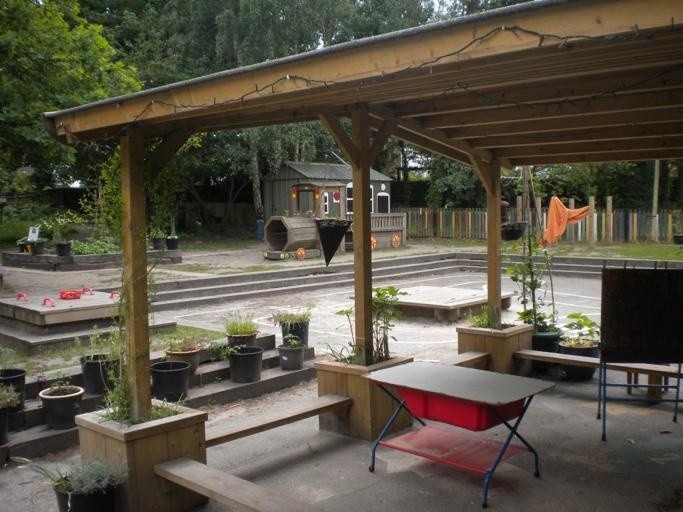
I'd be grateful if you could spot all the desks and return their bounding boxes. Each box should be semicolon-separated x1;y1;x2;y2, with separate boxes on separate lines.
362;361;556;508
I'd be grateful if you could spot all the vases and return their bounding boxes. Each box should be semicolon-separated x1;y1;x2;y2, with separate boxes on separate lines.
149;360;191;402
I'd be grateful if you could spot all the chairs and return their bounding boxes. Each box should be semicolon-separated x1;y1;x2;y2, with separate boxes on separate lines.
597;269;683;441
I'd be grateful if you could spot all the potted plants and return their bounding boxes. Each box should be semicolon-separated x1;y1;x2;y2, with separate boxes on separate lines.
502;239;564;371
165;335;202;373
0;345;27;411
16;236;28;252
56;236;72;256
208;343;265;383
224;309;257;346
0;383;22;446
278;334;306;371
32;239;45;254
269;301;313;347
11;454;129;512
147;225;167;249
557;313;600;381
167;235;178;250
38;369;84;429
75;325;119;394
